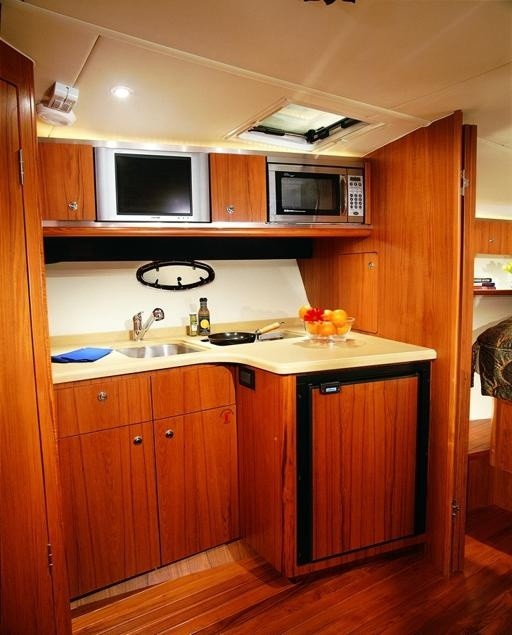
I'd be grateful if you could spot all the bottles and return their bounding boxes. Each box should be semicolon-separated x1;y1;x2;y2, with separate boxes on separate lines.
189;298;210;336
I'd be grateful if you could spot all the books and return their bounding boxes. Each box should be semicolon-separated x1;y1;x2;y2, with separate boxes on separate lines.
474;277;497;289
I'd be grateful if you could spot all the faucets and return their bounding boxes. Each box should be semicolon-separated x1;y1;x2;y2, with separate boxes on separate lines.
133;308;164;340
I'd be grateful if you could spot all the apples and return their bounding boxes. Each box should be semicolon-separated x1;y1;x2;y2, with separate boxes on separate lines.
503;263;512;274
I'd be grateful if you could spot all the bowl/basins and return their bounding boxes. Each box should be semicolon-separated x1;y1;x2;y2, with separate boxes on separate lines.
300;317;355;343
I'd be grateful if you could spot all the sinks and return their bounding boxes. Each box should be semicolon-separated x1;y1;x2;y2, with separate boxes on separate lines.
114;343;212;359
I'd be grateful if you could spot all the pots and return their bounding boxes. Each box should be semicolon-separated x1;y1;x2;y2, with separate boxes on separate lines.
208;321;288;346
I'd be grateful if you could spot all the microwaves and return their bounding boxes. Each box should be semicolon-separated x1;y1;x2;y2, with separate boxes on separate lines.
266;164;365;224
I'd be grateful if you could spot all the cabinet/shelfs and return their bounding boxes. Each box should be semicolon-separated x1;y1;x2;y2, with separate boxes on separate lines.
207;153;269;231
54;365;245;602
36;141;96;231
284;361;433;579
474;217;512;254
